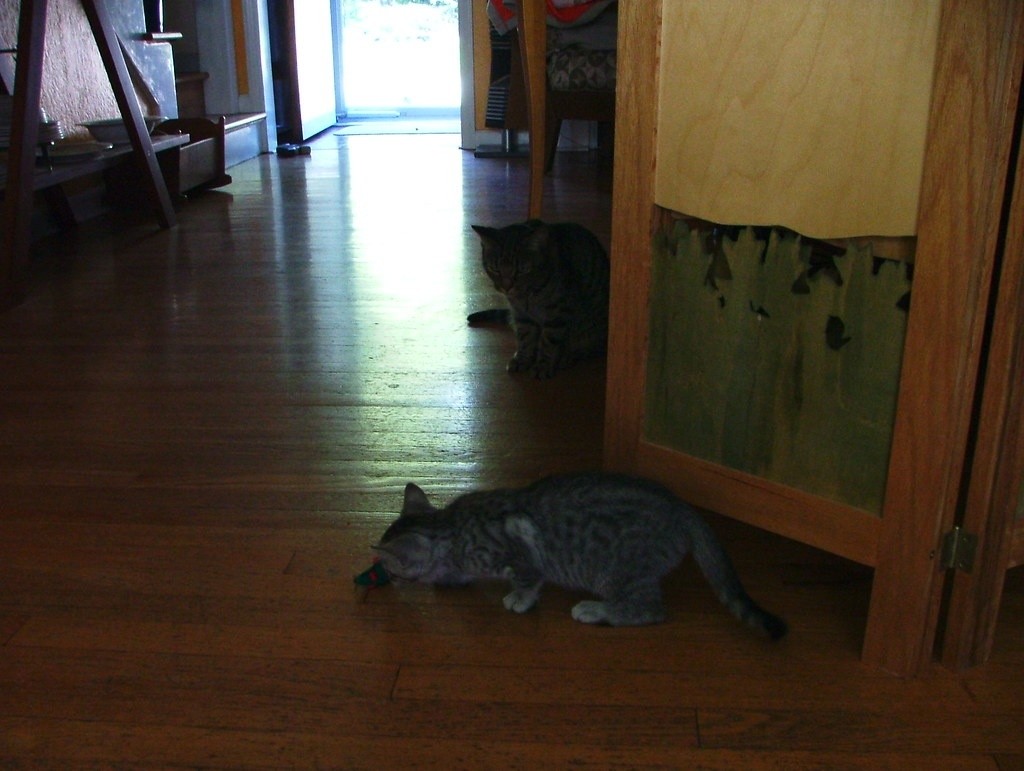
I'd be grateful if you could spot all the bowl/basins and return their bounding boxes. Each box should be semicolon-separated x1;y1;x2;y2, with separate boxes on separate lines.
75;115;169;144
35;141;113;164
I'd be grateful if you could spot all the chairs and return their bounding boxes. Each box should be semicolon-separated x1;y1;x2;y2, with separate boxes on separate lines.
505;0;618;222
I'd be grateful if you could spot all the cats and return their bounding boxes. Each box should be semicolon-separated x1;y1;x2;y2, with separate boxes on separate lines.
379;470;791;641
467;218;610;382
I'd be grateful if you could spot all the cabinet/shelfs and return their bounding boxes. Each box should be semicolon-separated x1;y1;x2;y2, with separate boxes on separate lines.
0;0;266;242
